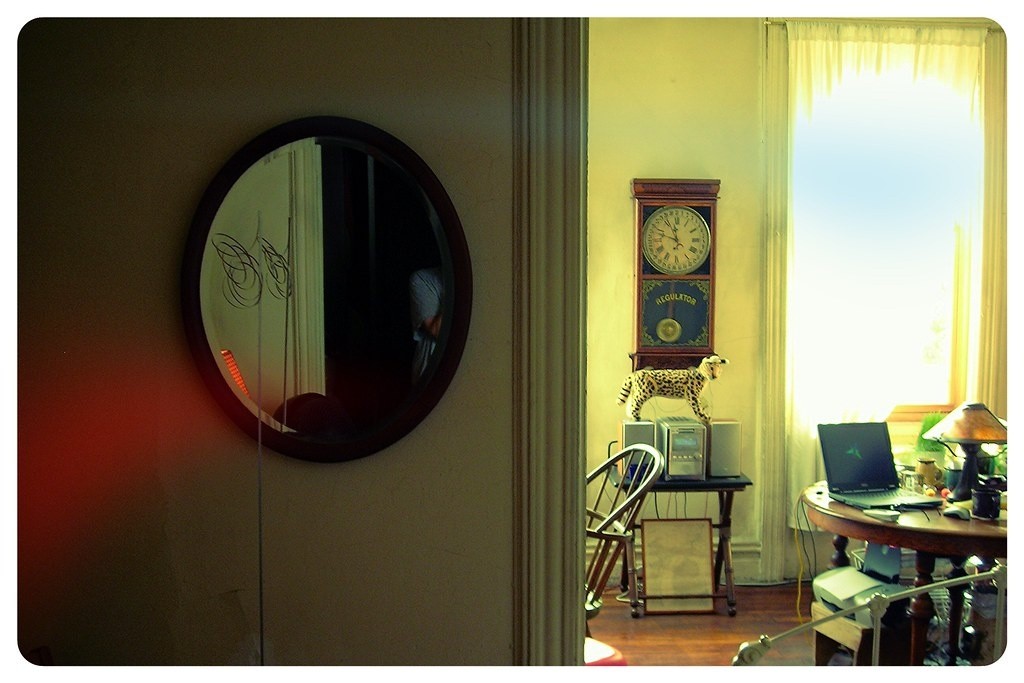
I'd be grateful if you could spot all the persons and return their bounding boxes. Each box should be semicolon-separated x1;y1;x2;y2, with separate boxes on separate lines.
273;393;350;439
407;262;472;402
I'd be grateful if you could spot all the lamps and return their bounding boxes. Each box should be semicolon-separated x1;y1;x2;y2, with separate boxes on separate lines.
923;398;1007;503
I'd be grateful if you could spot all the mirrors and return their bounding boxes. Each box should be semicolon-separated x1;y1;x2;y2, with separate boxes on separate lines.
179;115;475;464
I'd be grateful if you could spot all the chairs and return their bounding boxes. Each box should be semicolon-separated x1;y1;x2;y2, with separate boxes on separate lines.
585;444;665;639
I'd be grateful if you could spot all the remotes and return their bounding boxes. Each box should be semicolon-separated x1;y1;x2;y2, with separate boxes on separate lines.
863;509;901;521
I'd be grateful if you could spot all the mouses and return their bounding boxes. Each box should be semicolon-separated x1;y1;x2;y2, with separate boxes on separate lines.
942;507;970;521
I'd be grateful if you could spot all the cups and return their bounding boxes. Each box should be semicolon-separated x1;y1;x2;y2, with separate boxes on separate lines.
917;458;936;484
945;469;962;489
971;489;1001;519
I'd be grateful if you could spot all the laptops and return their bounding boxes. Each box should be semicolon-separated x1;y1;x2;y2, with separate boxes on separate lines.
818;422;944;510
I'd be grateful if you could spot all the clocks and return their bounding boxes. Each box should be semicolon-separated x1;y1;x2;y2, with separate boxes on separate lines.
629;177;721;371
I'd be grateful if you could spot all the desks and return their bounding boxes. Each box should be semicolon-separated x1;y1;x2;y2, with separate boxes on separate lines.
604;461;752;618
799;465;1007;666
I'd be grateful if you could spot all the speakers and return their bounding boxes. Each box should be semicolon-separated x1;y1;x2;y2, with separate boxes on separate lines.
621;419;656;476
705;418;740;476
